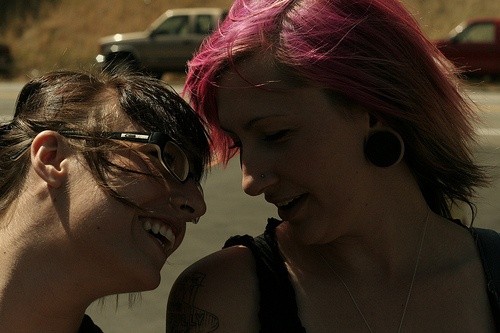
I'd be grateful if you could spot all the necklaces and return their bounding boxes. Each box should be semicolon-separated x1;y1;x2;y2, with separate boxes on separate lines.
315;205;431;333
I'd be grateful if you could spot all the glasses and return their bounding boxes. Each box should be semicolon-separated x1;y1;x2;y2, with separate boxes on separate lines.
10;130;204;224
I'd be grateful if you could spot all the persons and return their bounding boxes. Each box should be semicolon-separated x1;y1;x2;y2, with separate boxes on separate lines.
0;69;214;333
165;1;500;333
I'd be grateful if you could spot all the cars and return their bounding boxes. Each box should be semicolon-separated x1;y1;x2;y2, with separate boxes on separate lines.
429;15;500;83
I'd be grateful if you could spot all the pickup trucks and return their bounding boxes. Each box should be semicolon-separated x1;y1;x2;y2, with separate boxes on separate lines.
96;7;227;79
